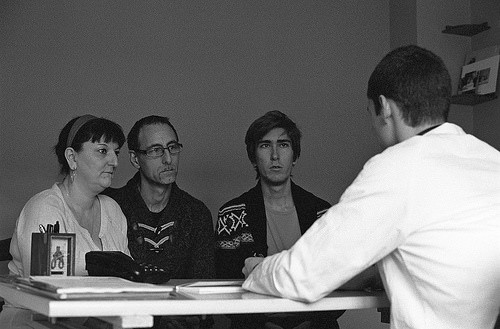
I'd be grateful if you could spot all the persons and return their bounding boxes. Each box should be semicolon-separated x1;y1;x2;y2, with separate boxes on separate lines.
0;114;136;329
215;110;347;329
242;44;500;328
99;115;218;329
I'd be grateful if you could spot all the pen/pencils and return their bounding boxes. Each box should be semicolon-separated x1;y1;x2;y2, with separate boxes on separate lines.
39;220;60;234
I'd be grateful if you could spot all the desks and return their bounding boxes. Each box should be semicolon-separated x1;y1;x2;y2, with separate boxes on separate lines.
0;278;390;329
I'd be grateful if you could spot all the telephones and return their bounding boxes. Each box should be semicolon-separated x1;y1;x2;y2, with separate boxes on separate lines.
86;250;171;284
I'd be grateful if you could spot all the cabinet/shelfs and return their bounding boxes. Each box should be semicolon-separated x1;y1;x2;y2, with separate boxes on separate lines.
442;22;497;106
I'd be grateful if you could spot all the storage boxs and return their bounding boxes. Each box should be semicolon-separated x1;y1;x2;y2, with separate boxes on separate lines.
30;233;76;276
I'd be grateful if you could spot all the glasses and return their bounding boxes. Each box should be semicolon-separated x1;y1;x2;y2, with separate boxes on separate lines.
135;143;183;157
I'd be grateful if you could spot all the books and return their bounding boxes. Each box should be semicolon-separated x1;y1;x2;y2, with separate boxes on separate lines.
176;278;243;293
15;276;176;301
